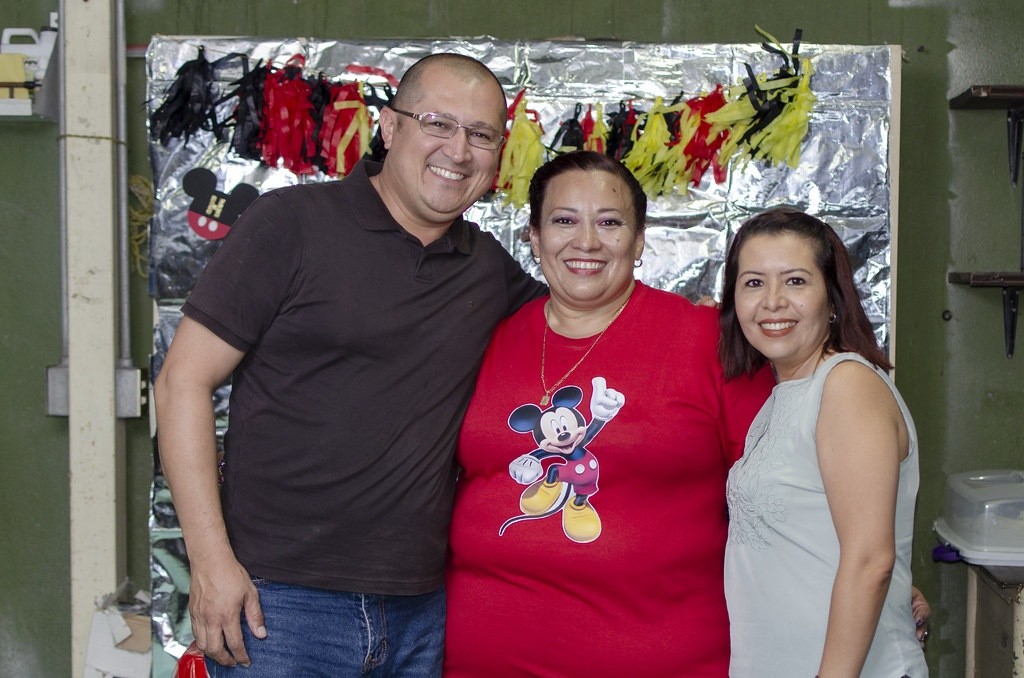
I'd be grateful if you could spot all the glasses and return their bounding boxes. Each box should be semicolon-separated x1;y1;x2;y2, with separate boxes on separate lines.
393;109;506;150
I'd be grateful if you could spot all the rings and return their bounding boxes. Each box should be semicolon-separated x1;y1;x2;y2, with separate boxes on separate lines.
923;631;927;640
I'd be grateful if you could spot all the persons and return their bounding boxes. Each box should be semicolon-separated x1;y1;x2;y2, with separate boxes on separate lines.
718;210;929;678
444;149;933;678
152;54;727;678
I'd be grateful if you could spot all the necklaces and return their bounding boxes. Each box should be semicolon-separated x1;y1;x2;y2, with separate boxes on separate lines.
541;297;629;406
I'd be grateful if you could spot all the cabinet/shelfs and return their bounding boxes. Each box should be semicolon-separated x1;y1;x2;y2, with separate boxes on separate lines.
948;85;1024;358
